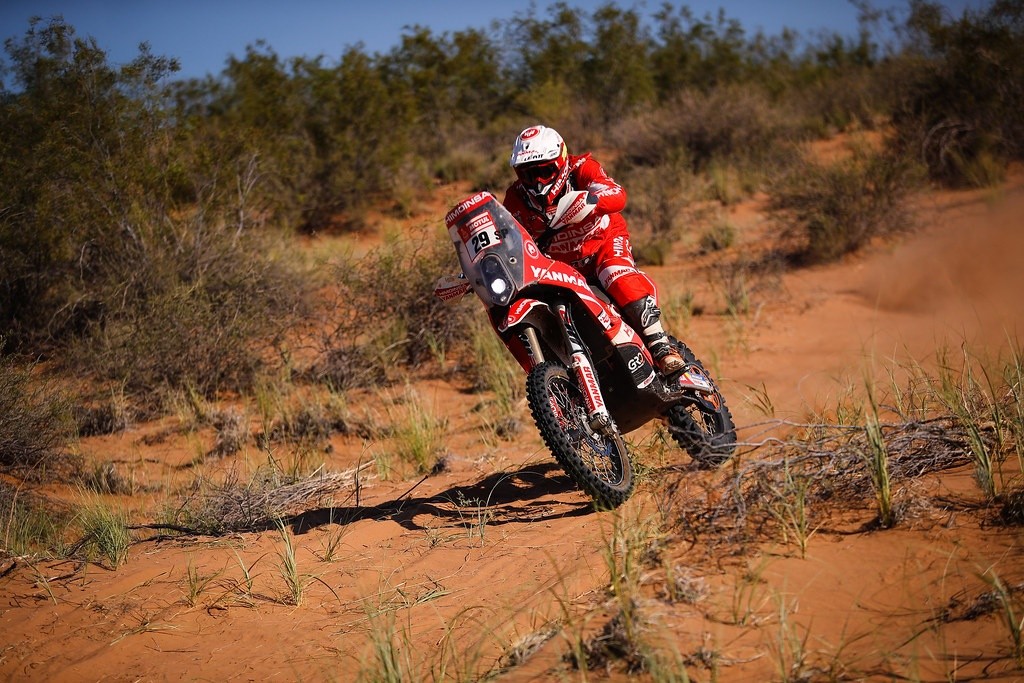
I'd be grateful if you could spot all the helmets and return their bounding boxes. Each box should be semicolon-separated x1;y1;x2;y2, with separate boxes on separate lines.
509;125;569;207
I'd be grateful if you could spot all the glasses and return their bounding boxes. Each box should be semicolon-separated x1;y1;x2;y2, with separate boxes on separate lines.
515;161;560;187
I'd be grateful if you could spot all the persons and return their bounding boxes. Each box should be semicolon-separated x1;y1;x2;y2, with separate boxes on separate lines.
501;124;687;377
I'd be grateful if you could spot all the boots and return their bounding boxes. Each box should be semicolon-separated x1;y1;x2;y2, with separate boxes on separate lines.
620;294;686;373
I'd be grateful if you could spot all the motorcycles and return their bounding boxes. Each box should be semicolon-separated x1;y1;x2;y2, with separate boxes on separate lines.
437;190;738;501
